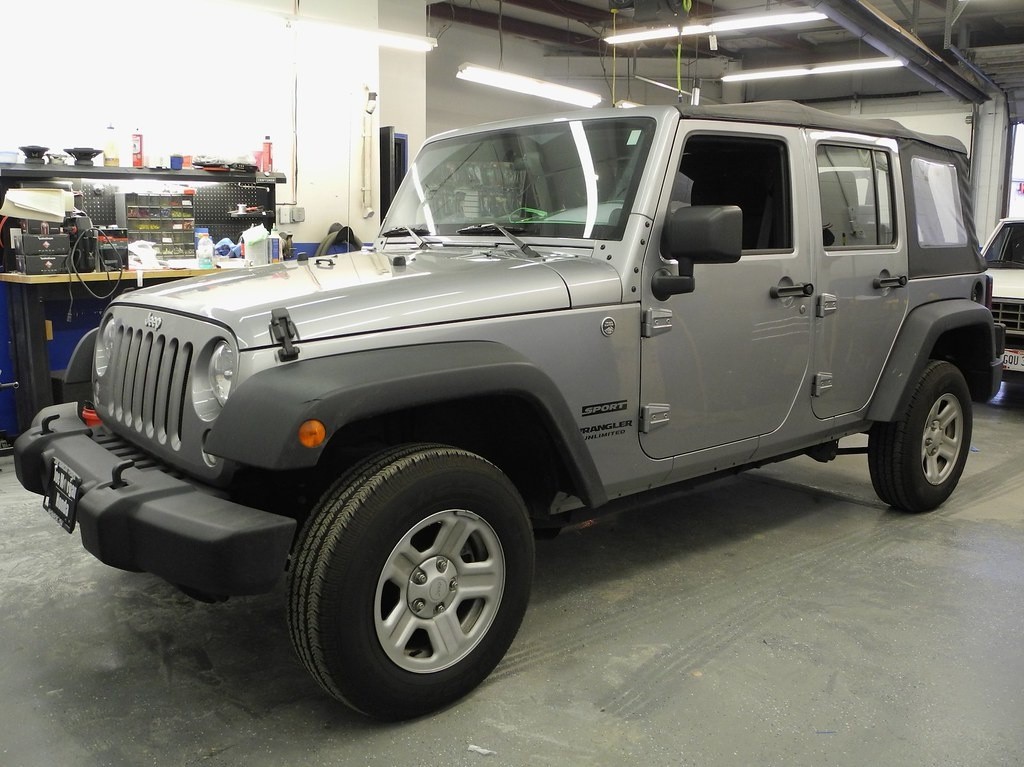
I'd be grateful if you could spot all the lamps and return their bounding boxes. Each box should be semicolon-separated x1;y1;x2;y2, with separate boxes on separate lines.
455;62;603;109
721;55;908;83
602;6;829;45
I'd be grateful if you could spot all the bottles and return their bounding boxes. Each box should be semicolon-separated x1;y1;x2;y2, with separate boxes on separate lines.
198;234;213;268
268;229;282;263
104;127;119;166
263;136;273;172
132;129;143;167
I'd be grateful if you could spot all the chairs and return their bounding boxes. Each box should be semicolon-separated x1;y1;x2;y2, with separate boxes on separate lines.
690;157;780;251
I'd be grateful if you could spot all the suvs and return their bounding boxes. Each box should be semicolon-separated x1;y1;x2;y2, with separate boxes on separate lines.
982;219;1024;382
15;99;1003;721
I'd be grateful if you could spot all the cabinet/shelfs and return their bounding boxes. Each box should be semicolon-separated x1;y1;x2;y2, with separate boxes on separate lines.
0;163;288;436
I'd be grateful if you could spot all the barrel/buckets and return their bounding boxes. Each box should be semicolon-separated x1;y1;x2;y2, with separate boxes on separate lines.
83;406;102;426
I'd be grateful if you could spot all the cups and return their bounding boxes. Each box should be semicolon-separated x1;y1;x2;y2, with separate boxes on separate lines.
171;157;183;170
239;205;246;213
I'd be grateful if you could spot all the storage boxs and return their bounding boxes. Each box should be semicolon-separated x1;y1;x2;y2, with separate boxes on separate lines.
13;219;74;275
